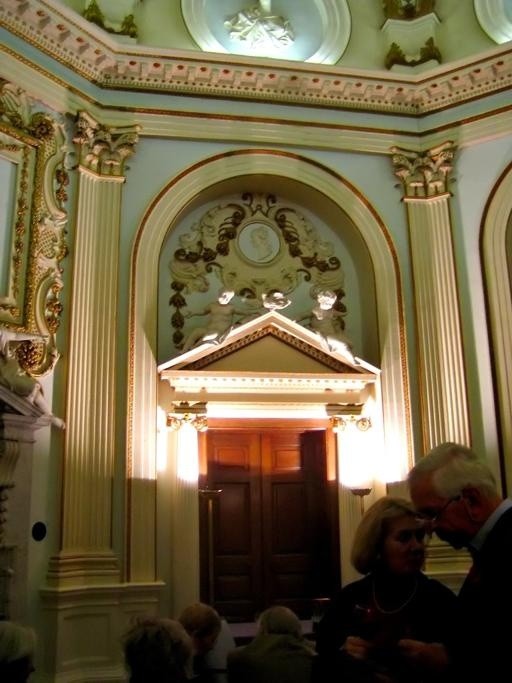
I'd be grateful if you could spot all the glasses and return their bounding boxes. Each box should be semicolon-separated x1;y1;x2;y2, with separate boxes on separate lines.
423;495;455;526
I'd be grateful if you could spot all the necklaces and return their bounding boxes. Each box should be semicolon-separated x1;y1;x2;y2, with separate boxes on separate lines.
369;575;420;614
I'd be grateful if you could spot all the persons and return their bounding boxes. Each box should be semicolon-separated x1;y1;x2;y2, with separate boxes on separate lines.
1;619;41;682
178;600;223;683
314;494;464;682
292;286;361;367
225;605;318;683
121;615;199;680
392;441;510;681
171;284;266;350
